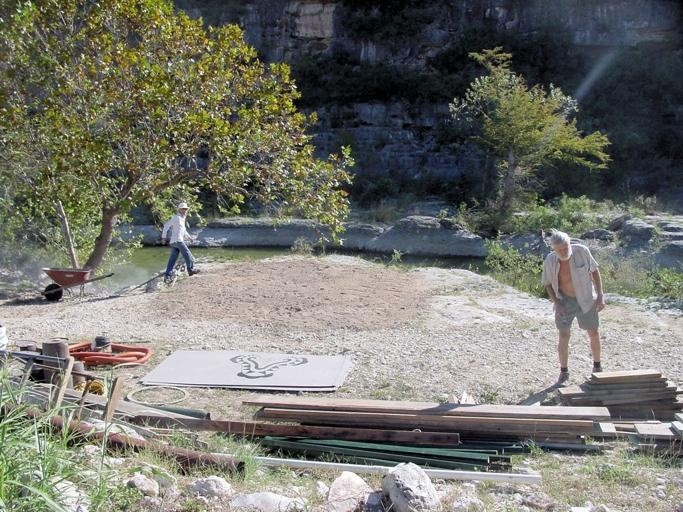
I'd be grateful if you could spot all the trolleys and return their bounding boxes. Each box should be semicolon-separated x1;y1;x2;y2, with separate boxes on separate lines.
38;265;117;304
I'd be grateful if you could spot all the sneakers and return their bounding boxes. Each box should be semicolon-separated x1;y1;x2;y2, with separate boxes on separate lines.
189;269;200;276
593;367;602;372
557;372;570;387
164;279;173;284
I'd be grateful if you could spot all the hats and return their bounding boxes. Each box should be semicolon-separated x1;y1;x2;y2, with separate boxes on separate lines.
178;202;189;208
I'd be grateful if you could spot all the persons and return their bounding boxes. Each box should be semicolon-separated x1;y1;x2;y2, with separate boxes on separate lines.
162;203;200;283
542;231;605;387
